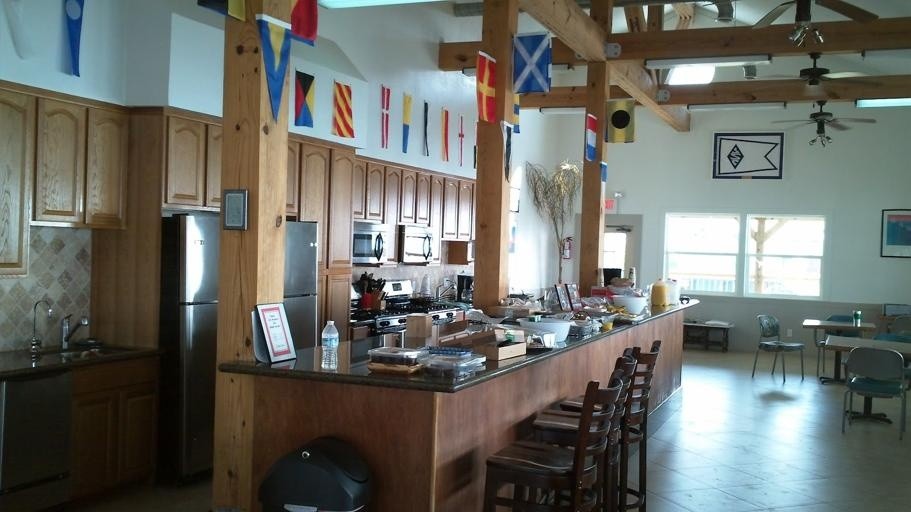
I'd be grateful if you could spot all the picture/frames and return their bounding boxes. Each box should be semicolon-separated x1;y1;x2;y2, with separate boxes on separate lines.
880;207;910;258
222;189;248;230
256;302;296;364
711;131;783;181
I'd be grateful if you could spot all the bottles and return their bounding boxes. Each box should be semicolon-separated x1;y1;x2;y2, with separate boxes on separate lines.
853;310;861;328
319;321;338;370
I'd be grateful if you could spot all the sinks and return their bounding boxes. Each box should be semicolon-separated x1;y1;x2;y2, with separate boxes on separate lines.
56;346;124;362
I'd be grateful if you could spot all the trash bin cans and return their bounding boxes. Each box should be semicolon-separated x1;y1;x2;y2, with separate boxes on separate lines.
257;433;378;512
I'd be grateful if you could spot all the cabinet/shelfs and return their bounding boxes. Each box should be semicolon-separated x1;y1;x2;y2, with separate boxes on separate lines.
32;96;126;224
444;178;472;240
417;173;444;227
126;112;222;206
472;183;476;242
354;158;383;224
317;270;351;344
384;165;417;226
287;141;299;214
68;355;162;493
299;141;353;270
0;79;32;278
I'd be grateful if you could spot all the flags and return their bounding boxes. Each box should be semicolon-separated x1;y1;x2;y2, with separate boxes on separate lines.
586;113;598;161
65;1;85;77
474;120;478;170
196;0;248;23
424;99;432;156
295;69;315;128
333;79;356;139
605;98;636;144
255;14;292;127
382;84;391;149
477;51;496;124
459;113;465;167
600;162;608;195
442;108;450;162
403;91;416;153
291;1;319;46
512;93;521;133
513;31;552;94
500;121;515;183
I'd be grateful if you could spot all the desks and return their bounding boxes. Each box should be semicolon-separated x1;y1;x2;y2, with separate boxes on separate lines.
684;320;734;354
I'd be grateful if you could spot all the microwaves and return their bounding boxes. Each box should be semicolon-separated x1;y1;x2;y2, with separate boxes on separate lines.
398;224;431;264
353;221;388;263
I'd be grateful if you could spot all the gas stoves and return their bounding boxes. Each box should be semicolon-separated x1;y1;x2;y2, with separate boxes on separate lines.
357;301;459;317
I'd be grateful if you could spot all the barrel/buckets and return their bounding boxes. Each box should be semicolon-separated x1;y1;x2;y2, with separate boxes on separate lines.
668;279;680;304
652;278;668;307
665;278;672;284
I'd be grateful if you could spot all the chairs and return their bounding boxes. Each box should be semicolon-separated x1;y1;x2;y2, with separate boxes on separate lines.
480;338;663;511
750;311;804;386
802;302;911;442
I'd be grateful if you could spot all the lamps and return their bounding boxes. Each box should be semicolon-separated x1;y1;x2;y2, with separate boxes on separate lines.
786;21;824;49
809;133;834;150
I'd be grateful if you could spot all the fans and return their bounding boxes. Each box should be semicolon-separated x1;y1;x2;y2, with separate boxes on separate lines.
757;53;870;99
744;0;876;28
769;100;876;132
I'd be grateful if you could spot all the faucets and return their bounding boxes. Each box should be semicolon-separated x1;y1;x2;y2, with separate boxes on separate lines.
436;283;455;302
30;300;52;354
59;313;89;350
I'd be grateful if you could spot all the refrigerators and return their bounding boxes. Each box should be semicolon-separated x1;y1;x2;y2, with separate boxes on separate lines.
284;220;317;347
163;212;221;484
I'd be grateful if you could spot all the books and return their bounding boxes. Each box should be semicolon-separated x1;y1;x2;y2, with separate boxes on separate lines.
366;342;527;374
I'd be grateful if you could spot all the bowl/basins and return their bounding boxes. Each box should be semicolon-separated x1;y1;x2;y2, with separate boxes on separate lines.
611;294;646;315
516;317;572;342
